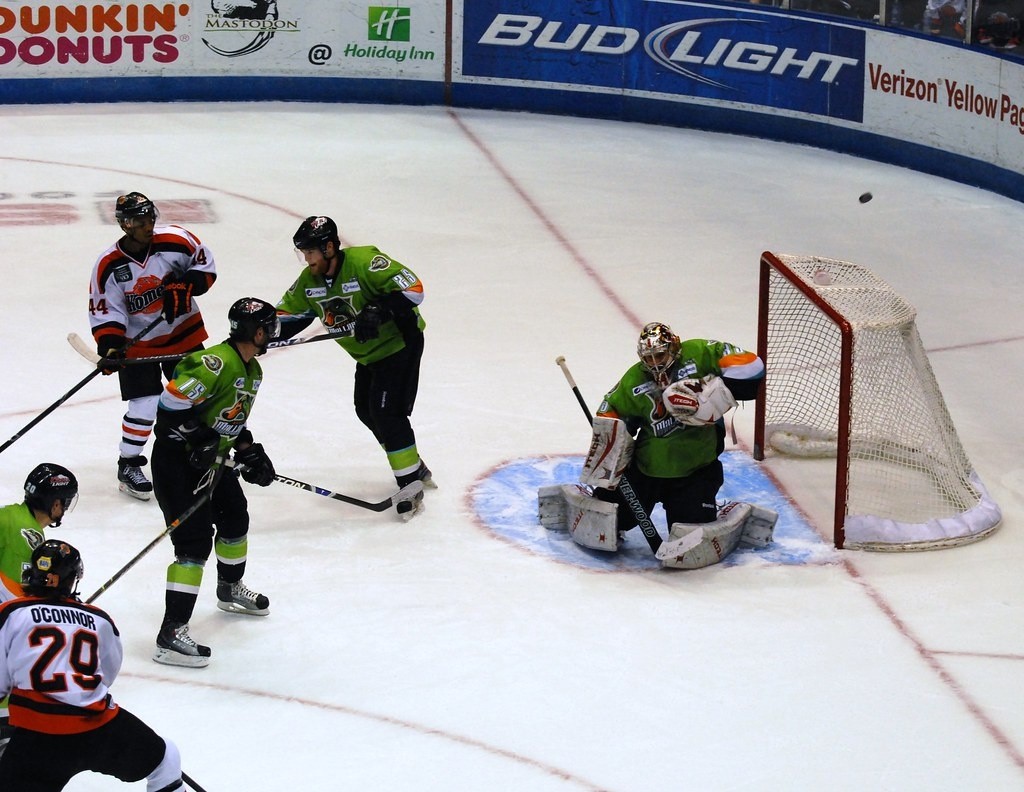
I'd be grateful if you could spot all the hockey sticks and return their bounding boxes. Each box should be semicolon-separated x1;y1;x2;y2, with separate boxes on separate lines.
555;355;662;556
82;483;216;605
66;330;356;368
215;455;426;513
0;312;167;454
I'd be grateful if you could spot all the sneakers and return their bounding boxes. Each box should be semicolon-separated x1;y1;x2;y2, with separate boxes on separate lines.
216;579;271;617
395;461;438;521
118;454;153;501
151;622;212;667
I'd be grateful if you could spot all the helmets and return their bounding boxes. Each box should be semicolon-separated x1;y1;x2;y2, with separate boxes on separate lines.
24;463;79;513
115;192;155;236
292;215;337;262
228;297;279;342
22;539;84;593
637;322;683;384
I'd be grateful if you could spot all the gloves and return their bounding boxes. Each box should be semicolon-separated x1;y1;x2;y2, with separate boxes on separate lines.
161;279;194;325
96;333;127;376
353;295;394;344
234;443;276;487
169;418;220;467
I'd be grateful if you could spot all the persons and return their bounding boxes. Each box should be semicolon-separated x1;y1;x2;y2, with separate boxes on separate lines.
151;298;279;656
0;462;209;792
88;192;217;492
255;217;432;514
537;322;780;569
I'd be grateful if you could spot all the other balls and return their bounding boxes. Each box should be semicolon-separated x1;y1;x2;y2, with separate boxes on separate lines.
859;192;872;203
